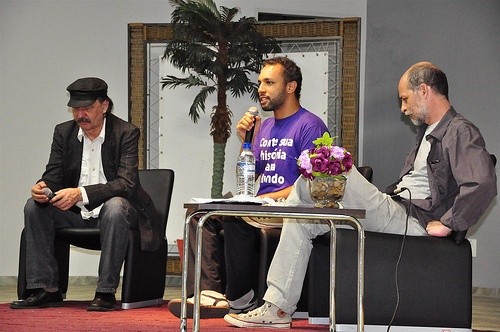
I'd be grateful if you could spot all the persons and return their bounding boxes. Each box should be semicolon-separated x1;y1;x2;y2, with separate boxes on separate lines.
225;61;497;330
168;57;330;319
8;78;160;311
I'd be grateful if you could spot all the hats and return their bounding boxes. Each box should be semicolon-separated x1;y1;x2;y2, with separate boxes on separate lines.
66;78;108;107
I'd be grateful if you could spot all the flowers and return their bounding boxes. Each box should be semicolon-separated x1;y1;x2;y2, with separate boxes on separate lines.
295;130;355;183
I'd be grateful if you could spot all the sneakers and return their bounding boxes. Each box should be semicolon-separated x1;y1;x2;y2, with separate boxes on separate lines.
226;293;260;315
224;300;293;329
167;289;230;319
241;196;285;229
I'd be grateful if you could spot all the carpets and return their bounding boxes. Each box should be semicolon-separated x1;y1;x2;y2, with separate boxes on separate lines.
0;300;333;332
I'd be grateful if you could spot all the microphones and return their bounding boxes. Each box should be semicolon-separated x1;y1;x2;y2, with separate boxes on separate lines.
244;107;259;143
386;187;406;195
42;188;81;215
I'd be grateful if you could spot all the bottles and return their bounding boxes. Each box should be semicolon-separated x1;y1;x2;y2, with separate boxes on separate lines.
236;143;255;197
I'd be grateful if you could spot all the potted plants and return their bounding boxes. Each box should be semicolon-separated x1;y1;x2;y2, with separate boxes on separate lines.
158;0;283;265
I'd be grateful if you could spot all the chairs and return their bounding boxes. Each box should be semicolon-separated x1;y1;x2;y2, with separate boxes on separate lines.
218;152;498;332
16;168;174;310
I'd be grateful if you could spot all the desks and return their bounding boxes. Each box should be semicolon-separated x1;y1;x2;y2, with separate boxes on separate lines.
179;204;367;332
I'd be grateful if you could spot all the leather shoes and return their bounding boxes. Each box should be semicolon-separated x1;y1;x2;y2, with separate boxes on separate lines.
9;288;63;309
86;292;117;311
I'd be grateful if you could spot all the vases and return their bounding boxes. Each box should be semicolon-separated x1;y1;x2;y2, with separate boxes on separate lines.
309;177;348;208
